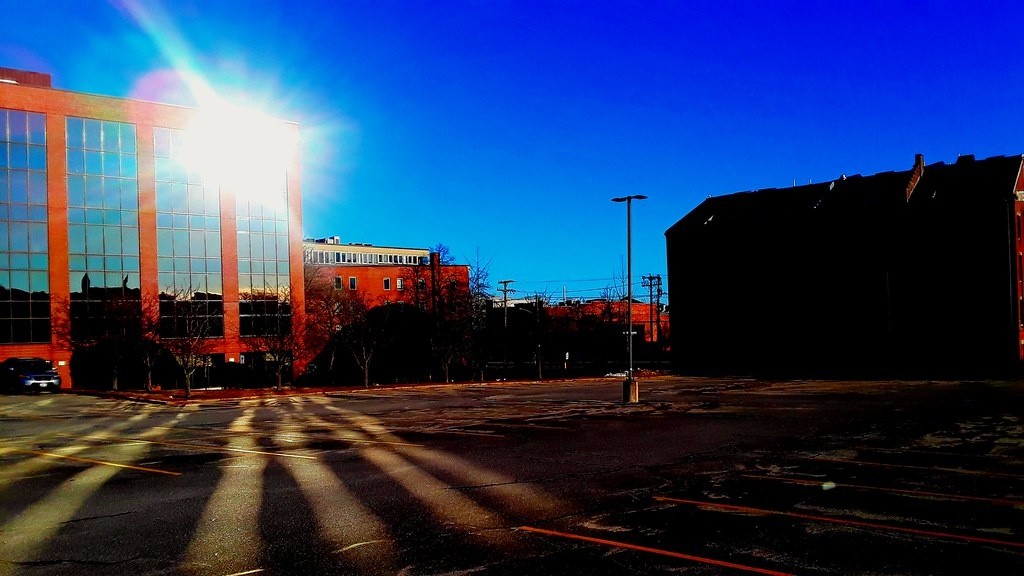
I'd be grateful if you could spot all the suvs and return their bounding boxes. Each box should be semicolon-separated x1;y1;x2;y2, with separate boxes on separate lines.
0;358;61;394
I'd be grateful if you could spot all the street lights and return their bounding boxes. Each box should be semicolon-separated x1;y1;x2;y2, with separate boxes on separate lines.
612;195;648;403
